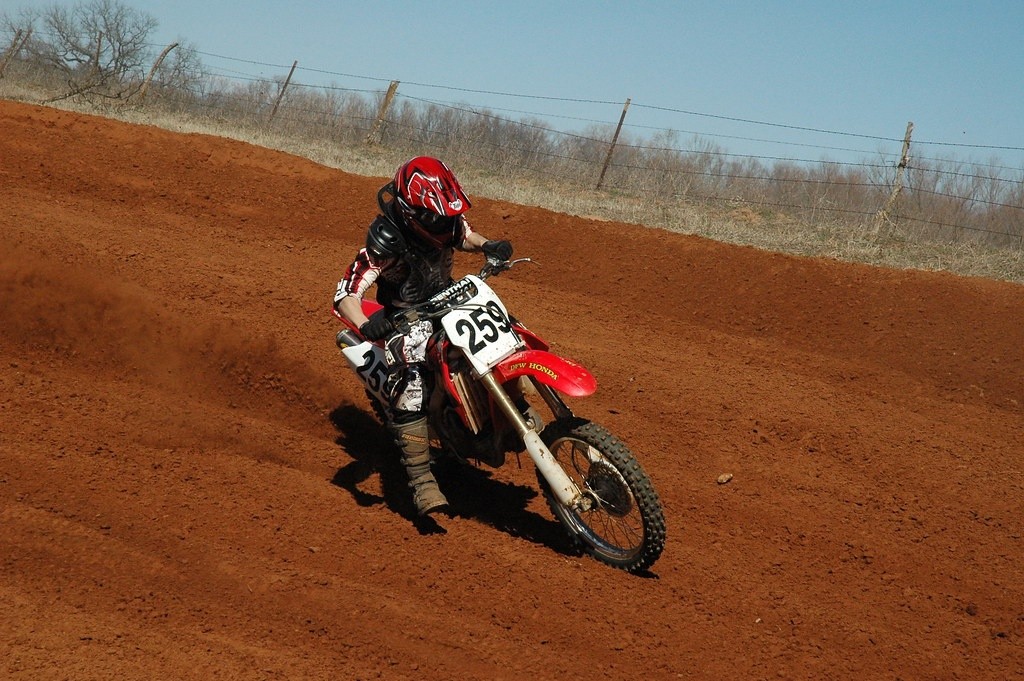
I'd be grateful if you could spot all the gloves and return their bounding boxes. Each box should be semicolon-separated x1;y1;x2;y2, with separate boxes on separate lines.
481;239;513;264
359;318;393;342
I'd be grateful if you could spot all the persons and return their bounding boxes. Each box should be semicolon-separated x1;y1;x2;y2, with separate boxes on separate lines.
333;154;515;517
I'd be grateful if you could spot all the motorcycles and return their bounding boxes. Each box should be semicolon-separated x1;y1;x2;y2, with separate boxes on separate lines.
331;239;668;575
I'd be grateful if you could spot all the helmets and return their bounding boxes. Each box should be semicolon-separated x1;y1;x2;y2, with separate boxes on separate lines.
392;156;472;251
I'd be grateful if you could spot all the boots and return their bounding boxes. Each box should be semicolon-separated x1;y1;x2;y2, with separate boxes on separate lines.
504;377;545;436
391;415;450;518
